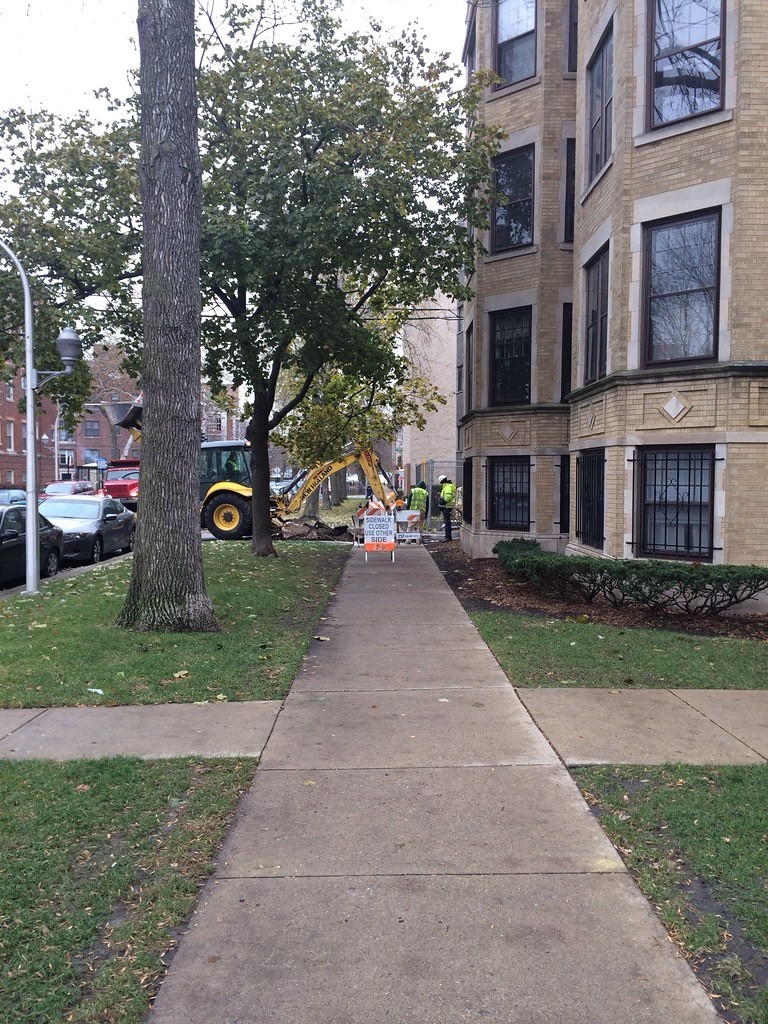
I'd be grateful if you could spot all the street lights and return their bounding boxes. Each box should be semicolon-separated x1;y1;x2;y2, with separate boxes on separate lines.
0;240;82;596
67;451;72;474
40;433;57;480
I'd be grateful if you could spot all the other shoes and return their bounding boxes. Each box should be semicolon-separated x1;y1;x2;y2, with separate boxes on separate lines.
416;541;420;544
440;538;452;543
405;541;411;544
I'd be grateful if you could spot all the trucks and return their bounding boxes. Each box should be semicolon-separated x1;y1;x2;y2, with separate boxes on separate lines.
95;459;141;511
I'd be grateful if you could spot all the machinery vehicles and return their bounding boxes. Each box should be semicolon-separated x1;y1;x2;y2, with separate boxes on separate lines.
98;402;406;541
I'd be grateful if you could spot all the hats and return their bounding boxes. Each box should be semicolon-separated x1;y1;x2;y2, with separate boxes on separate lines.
417;481;426;489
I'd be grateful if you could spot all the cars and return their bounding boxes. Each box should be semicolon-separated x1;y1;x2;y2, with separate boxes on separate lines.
269;475;298;494
296;477;307;488
37;495;136;564
284;476;293;482
0;489;43;506
0;504;64;588
37;480;94;505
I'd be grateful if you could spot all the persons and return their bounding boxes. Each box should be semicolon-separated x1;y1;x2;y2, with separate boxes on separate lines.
367;484;373;501
439;475;456;543
405;480;429;545
223;453;239;473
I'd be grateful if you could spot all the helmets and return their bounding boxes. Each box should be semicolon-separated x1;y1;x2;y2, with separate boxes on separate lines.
438;475;446;483
